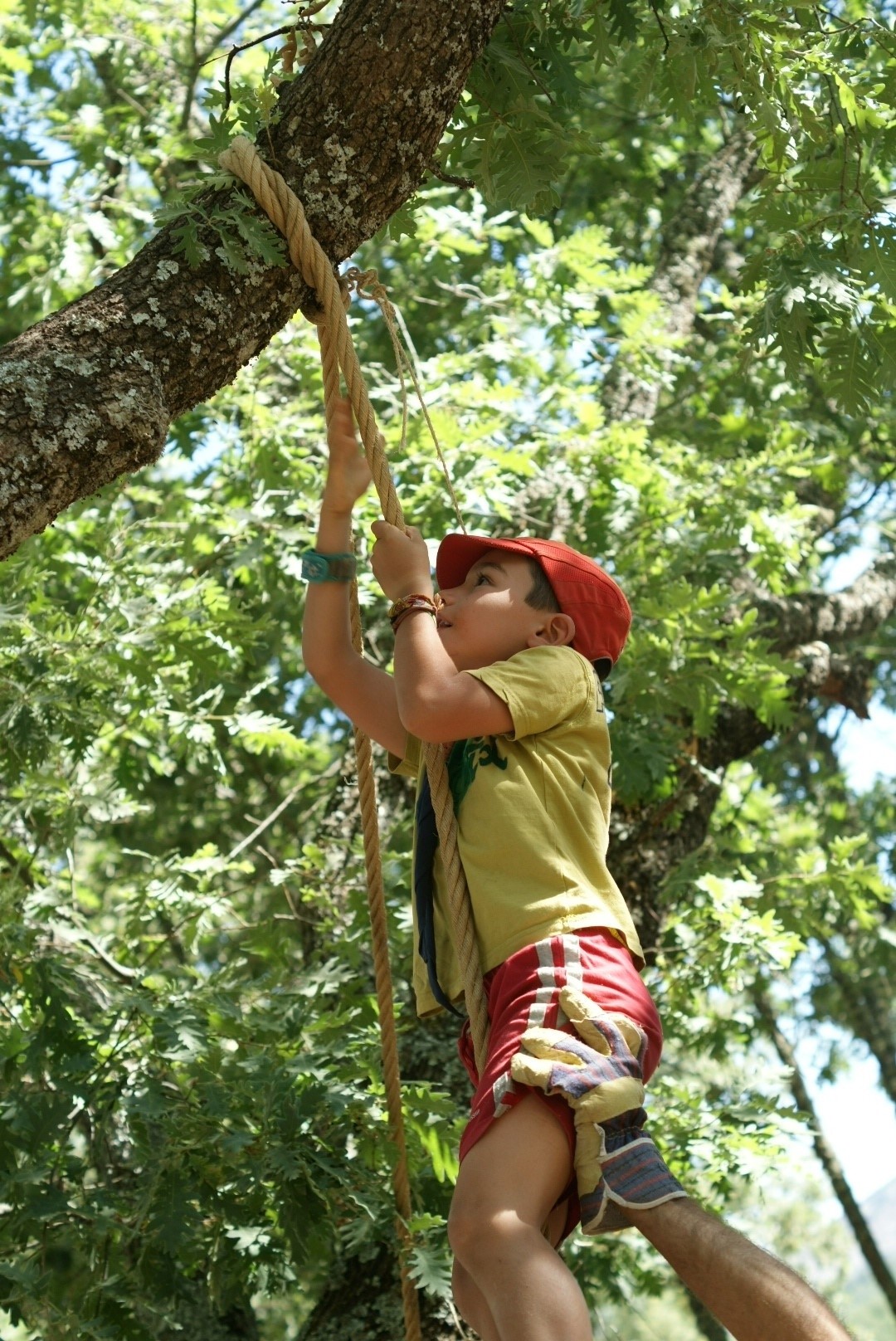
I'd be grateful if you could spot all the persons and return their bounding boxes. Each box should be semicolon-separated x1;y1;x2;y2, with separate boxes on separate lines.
302;397;660;1341
510;987;854;1341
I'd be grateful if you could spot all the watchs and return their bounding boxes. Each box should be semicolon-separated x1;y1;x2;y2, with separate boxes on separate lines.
300;546;357;583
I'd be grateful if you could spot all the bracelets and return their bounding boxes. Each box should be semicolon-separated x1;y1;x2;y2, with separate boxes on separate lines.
388;594;439;636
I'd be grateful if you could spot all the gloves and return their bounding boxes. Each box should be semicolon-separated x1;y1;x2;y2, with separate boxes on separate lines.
511;986;690;1236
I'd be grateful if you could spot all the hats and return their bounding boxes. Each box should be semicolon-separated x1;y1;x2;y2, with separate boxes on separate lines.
436;534;633;684
